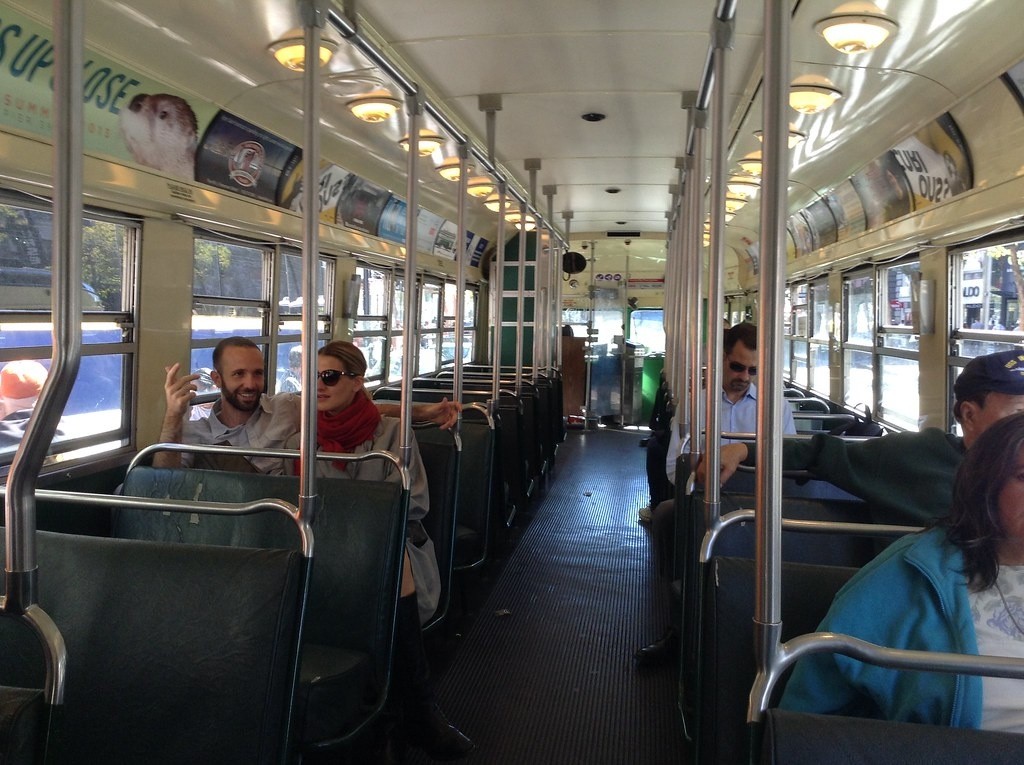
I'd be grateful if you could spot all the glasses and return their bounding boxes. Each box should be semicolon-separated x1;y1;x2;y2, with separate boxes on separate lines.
725;352;758;376
317;369;359;387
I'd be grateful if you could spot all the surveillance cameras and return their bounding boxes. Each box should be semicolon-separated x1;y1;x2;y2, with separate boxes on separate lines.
582;242;587;249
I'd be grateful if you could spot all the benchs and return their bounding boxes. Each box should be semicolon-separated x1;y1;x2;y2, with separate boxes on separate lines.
648;367;1024;764
0;358;565;765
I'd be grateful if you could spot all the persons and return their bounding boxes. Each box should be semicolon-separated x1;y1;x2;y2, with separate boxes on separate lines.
775;410;1024;739
635;319;796;663
187;367;222;421
696;350;1024;598
892;320;915;341
0;359;67;450
989;565;1024;635
284;341;476;765
962;316;1024;354
150;336;463;476
353;310;472;388
279;345;304;398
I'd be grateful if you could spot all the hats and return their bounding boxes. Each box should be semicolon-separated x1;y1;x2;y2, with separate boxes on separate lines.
961;350;1023;395
2;359;48;399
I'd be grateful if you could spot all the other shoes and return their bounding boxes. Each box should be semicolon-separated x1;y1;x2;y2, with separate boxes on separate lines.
640;437;652;445
636;618;683;666
639;506;653;523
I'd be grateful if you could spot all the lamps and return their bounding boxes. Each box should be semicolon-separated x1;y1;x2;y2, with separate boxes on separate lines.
268;29;333;75
813;1;901;57
437;158;474;180
345;82;400;123
700;73;845;247
483;193;514;211
396;128;445;159
464;175;494;197
501;210;525;223
516;217;536;230
